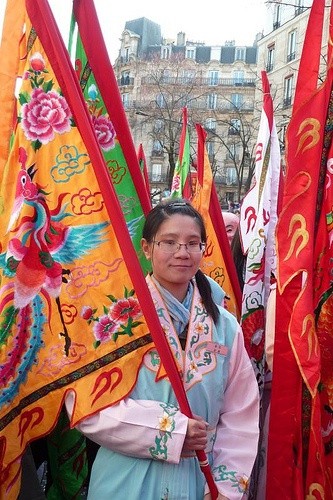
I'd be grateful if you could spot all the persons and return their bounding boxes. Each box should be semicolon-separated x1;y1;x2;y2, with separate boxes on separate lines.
64;197;260;500
220;212;240;245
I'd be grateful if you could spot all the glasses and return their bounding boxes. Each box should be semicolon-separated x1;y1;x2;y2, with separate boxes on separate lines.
148;240;204;252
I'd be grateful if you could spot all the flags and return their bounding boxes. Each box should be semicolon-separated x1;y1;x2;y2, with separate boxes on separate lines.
0;0;333;500
